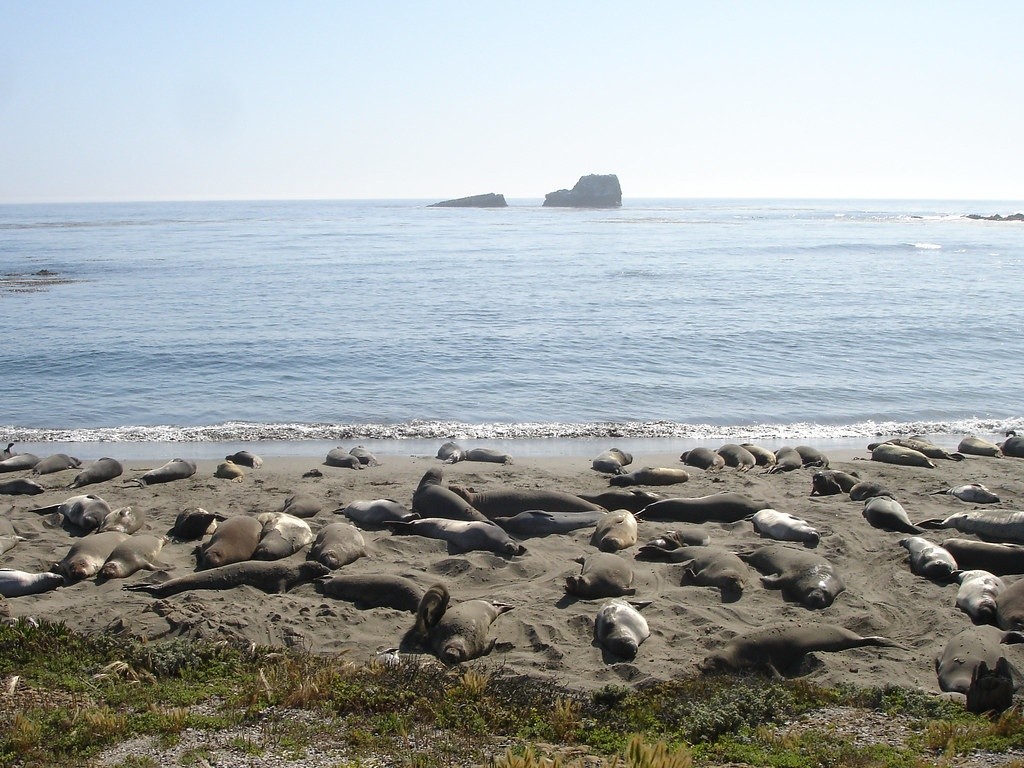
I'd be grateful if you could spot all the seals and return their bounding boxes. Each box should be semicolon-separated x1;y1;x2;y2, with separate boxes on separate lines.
1;429;1024;714
